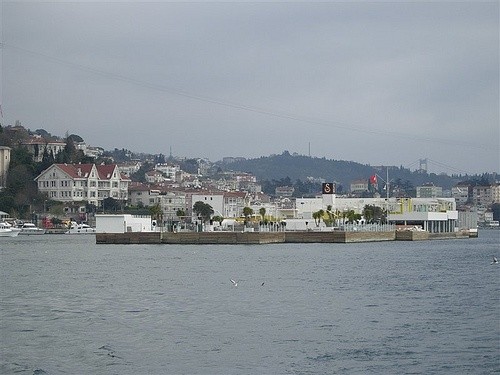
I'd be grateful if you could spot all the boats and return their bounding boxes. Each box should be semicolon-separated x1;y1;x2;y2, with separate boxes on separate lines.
0;218;95;237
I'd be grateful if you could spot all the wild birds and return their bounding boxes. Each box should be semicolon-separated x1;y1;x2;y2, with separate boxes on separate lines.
490;257;498;265
